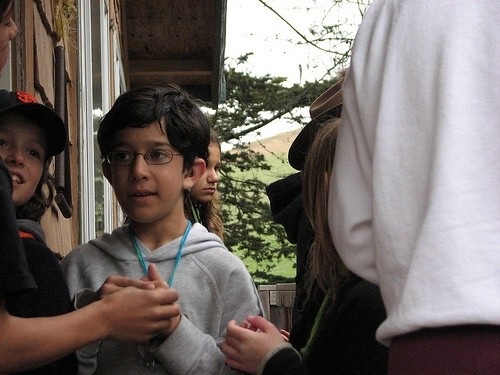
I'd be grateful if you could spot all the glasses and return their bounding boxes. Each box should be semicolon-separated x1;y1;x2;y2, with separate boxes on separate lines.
105;148;185;166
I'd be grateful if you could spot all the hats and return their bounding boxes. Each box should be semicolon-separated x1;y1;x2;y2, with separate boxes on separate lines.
288;75;345;172
0;88;68;156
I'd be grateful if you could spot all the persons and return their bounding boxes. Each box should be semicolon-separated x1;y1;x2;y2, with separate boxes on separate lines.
0;0;181;375
185;133;223;237
328;1;500;375
59;85;265;375
0;88;79;375
223;70;389;375
